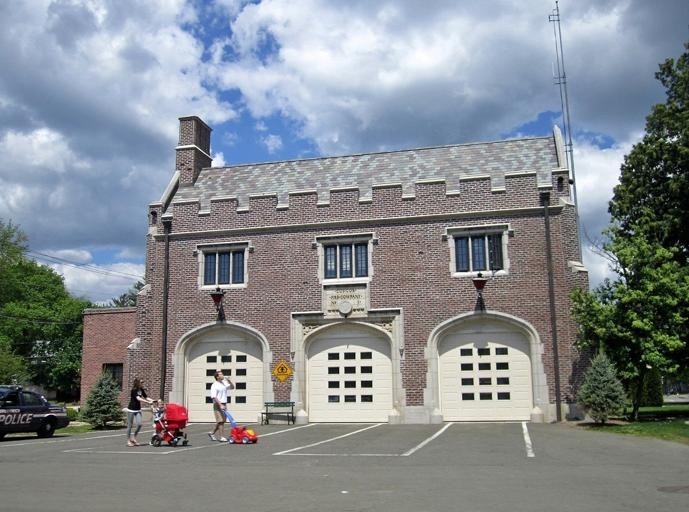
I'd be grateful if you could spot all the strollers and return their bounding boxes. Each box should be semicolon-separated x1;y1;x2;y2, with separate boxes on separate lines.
151;400;189;447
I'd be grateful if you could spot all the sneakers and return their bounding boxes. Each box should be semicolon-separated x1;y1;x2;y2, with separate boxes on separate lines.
126;438;140;447
217;435;228;442
208;432;218;441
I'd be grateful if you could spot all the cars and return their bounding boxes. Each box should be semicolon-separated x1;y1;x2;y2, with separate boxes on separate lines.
0;384;69;437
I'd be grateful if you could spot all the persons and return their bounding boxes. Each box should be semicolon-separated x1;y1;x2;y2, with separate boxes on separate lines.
149;402;164;446
126;376;153;447
207;370;235;442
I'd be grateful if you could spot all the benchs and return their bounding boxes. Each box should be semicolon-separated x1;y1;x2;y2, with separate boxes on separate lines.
261;402;295;425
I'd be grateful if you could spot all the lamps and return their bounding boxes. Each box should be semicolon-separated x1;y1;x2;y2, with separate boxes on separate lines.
471;271;488;311
208;287;227;322
